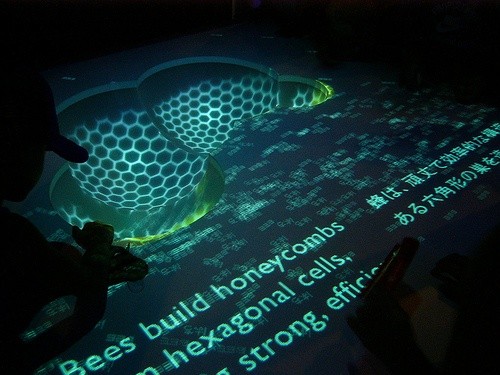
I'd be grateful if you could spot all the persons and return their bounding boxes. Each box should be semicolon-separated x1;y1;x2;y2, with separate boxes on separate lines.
394;263;479;370
